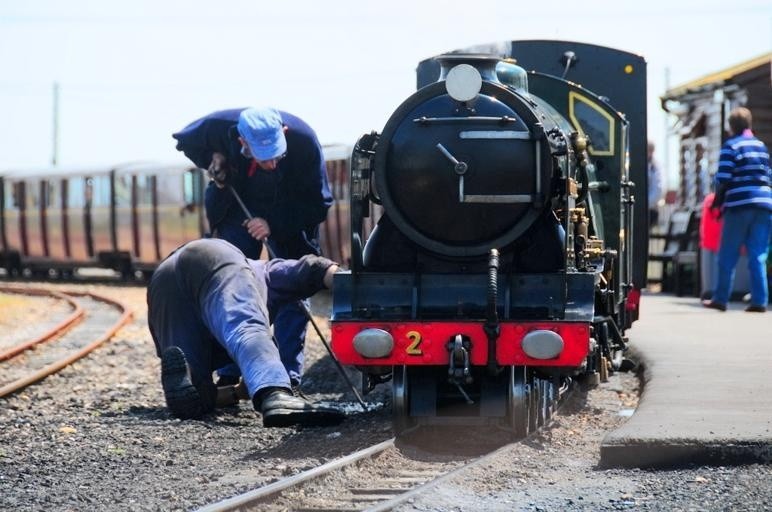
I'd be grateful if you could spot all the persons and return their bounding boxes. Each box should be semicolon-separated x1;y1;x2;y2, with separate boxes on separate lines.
644;140;663;233
172;106;335;401
696;177;723;301
703;105;771;314
144;234;348;427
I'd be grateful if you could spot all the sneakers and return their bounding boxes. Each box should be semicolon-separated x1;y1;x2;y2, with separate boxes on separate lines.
160;346;209;417
261;392;346;428
216;374;239;388
746;305;765;313
701;299;726;311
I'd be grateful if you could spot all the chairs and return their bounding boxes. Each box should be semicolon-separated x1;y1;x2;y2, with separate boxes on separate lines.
646;210;695;293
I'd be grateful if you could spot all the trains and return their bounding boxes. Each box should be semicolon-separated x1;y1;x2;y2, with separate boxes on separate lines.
0;38;650;441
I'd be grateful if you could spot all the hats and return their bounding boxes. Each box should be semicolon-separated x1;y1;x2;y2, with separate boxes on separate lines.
237;107;288;162
